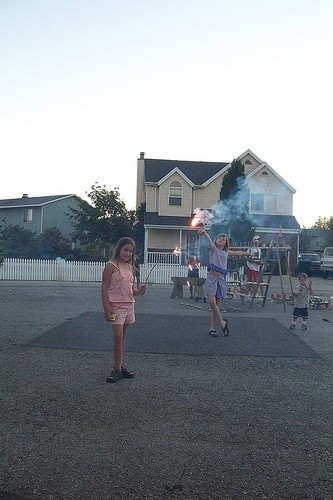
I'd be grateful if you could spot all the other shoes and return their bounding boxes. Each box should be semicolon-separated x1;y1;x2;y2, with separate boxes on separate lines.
106;368;134;382
290;322;295;329
301;323;307;330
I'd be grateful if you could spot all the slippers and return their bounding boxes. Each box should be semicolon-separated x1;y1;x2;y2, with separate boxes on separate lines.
222;319;228;336
209;328;217;337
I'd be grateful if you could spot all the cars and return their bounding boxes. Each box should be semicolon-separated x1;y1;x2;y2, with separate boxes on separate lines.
297;253;321;274
321;247;333;279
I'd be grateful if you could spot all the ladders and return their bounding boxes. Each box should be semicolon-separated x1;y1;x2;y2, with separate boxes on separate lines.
248;233;296;313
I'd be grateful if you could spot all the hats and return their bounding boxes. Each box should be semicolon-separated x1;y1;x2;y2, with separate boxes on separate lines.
253;235;260;241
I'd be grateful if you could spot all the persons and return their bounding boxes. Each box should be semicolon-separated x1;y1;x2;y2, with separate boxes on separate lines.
246;235;268;297
187;248;201;299
197;228;253;338
133;249;143;291
101;237;148;383
289;273;313;330
